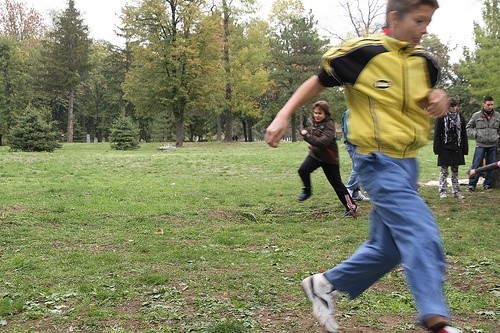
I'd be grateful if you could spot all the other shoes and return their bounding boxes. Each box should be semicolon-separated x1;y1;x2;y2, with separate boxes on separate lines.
298;194;309;201
440;190;447;200
345;206;359;216
452;192;465;199
466;188;474;192
347;188;370;202
484;186;493;191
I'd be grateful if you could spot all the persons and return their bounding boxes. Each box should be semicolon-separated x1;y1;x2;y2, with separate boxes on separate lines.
433;97;468;199
341;109;371;201
470;161;500;176
298;100;356;216
265;0;465;332
466;96;500;192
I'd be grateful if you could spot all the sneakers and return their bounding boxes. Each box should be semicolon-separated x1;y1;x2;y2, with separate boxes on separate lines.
438;325;463;333
301;273;341;333
